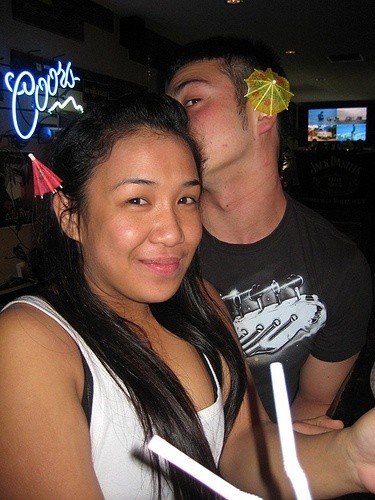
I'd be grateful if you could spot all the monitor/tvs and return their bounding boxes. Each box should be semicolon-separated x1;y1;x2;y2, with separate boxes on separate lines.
298;99;375;150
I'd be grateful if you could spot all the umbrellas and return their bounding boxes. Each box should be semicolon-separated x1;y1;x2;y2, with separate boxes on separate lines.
28;152;63;199
244;67;295;117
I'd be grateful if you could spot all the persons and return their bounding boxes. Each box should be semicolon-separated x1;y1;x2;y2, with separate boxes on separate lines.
0;35;375;500
316;111;357;138
